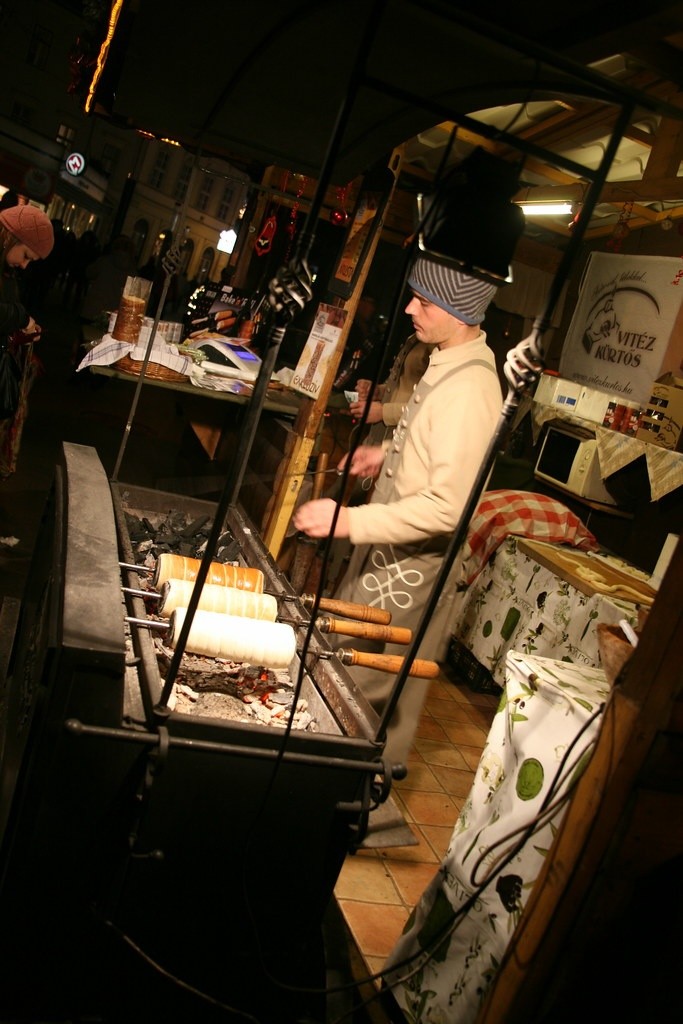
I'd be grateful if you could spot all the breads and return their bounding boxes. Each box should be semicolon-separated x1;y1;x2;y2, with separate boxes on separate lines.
170;607;296;668
158;579;278;623
155;553;265;593
112;295;146;345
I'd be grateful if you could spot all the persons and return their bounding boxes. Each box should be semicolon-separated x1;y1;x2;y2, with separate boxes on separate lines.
0;204;55;483
291;249;503;773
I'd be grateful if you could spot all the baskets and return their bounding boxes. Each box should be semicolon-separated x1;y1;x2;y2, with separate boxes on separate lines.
115;351;197;383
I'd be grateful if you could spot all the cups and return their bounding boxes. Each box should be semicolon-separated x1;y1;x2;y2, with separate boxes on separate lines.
531;373;645;437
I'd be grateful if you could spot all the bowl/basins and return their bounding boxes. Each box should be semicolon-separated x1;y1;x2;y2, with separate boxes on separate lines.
593;621;643;690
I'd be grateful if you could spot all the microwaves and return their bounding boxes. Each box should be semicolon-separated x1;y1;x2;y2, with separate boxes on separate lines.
533;427;626;507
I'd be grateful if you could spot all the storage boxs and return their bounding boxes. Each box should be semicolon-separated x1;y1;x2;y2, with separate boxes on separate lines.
635;371;683;451
447;637;502;697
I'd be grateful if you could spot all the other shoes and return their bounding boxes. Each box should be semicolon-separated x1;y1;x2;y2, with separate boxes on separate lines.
0;536;34;557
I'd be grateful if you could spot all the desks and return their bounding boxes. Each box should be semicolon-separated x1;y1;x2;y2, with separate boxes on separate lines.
83;341;300;498
445;534;658;690
378;649;611;1024
534;475;635;529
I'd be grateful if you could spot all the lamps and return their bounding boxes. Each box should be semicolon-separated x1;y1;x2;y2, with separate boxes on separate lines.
519;202;578;215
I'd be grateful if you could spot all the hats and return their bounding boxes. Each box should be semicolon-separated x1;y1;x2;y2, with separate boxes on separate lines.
0;203;55;260
407;251;506;327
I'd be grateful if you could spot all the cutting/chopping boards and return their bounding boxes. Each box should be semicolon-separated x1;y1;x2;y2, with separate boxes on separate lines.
514;537;660;602
